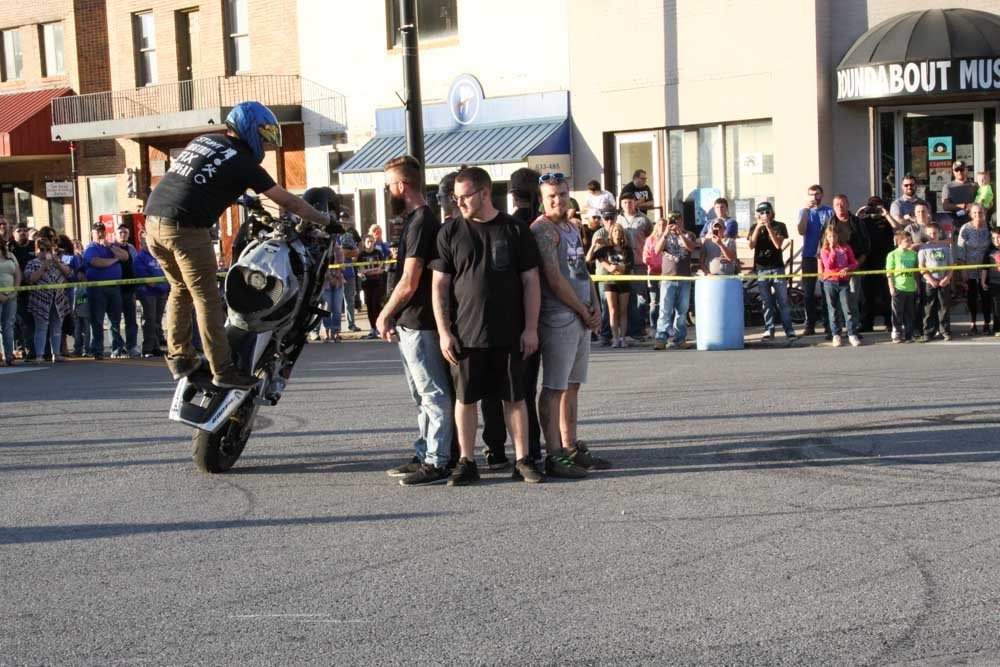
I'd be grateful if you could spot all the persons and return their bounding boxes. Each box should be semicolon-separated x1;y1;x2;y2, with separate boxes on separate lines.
747;202;797;341
798;162;1000;347
0;221;172;366
695;198;737;277
310;212;400;343
376;156;613;487
144;101;345;389
539;169;698;351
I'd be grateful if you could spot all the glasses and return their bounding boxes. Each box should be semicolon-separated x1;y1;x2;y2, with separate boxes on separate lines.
435;192;452;206
604;216;614;221
593;217;601;219
954;167;965;172
538;172;565;186
452;186;482;202
384;180;409;192
902;183;915;187
39;247;51;252
759;211;771;215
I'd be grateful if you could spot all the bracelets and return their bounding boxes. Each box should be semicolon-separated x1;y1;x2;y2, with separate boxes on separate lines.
663;235;667;240
108;243;113;250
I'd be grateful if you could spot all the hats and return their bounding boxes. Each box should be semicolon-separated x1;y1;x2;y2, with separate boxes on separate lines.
603;207;616;218
953;160;966;170
713;218;725;228
92;222;105;230
586;209;602;217
757;202;772;212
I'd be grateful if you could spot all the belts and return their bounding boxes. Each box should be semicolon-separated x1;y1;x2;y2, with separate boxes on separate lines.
155;215;197;229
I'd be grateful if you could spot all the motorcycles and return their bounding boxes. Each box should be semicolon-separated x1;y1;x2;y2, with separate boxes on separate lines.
167;186;345;474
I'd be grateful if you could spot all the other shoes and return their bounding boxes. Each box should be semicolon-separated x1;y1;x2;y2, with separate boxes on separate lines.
588;328;1000;350
171;354;202;381
212;363;262;389
0;338;168;367
307;319;379;344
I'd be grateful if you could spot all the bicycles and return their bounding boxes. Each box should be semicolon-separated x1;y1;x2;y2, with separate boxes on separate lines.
687;277;812;332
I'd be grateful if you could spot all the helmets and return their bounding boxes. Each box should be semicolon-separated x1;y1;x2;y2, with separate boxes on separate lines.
225;102;282;164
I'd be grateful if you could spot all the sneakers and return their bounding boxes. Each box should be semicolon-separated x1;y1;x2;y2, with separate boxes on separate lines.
386;434;612;489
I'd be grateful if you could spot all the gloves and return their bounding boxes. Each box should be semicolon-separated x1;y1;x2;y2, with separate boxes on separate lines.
326;216;344;236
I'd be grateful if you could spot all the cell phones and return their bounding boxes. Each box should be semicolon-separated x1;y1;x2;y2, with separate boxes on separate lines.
669;220;675;225
866;208;881;213
760;214;768;222
713;230;718;237
